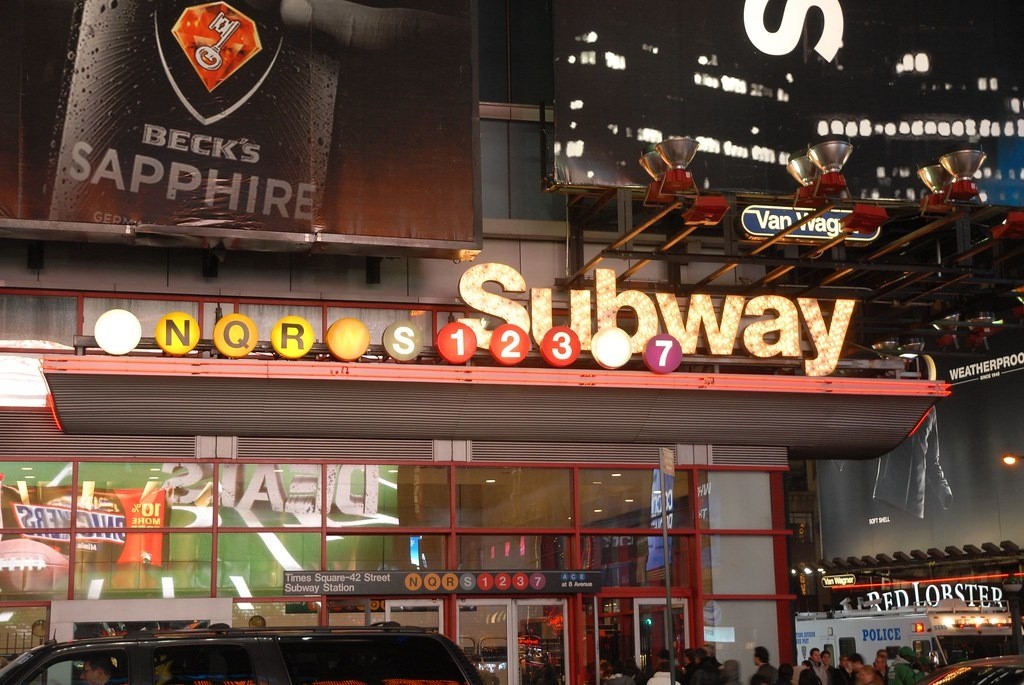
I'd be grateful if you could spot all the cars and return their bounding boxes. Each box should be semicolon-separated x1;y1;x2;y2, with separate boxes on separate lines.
914;654;1024;685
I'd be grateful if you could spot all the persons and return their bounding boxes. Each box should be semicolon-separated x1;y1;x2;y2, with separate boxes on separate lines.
599;644;927;685
80;656;122;685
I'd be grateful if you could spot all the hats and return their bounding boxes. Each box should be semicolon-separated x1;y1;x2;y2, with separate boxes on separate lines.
898;646;916;657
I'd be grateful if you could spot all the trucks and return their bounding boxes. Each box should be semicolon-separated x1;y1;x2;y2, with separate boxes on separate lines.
795;597;1024;669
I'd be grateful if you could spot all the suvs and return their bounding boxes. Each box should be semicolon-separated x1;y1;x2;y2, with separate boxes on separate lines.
0;624;487;685
